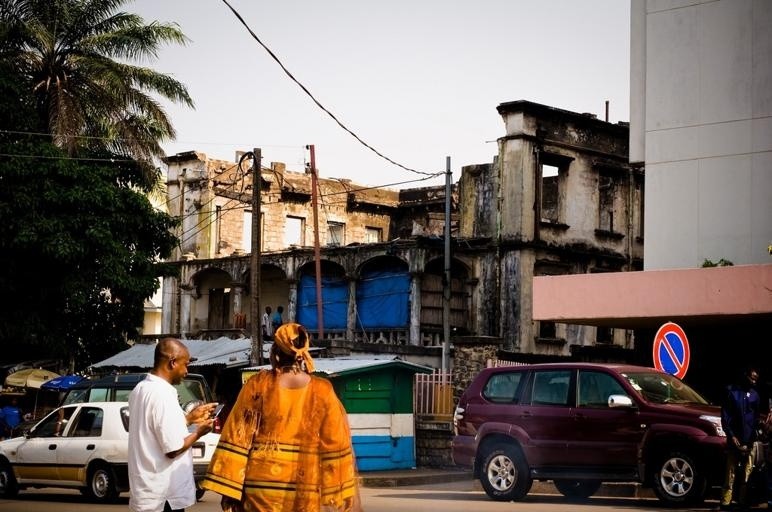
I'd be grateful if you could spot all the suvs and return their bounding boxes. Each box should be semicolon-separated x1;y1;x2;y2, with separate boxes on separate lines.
450;362;729;507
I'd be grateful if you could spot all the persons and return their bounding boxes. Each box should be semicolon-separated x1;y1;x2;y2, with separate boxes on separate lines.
0;400;24;439
271;306;284;330
708;366;772;512
261;306;273;341
198;322;363;512
128;338;220;512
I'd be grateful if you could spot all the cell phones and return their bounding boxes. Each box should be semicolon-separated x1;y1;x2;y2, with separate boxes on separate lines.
208;404;225;419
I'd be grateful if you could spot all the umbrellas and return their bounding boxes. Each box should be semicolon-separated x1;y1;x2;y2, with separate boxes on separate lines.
40;375;92;391
4;366;62;418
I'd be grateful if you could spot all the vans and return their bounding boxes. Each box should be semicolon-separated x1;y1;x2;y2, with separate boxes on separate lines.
60;371;221;432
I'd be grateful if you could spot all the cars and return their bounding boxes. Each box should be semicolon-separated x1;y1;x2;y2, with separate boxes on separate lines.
0;401;222;502
